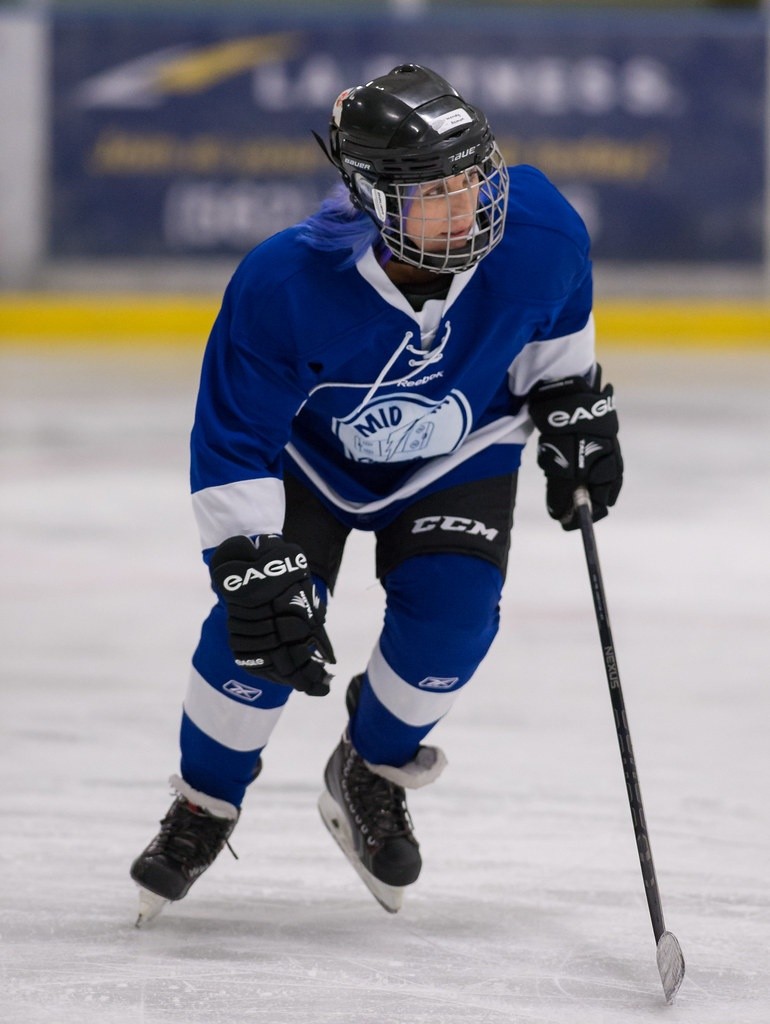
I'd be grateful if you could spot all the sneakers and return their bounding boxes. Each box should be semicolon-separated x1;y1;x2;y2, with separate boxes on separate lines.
316;727;449;915
129;758;261;930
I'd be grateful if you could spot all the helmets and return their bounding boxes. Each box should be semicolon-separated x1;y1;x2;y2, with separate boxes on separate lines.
313;62;495;219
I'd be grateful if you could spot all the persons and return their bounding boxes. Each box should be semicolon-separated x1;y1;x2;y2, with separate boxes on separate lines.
129;61;624;931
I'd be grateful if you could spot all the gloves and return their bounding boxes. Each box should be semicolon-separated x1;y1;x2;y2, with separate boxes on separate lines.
529;363;625;531
209;532;337;698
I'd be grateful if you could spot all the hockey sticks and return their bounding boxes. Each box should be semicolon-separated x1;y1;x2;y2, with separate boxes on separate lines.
573;485;688;1005
